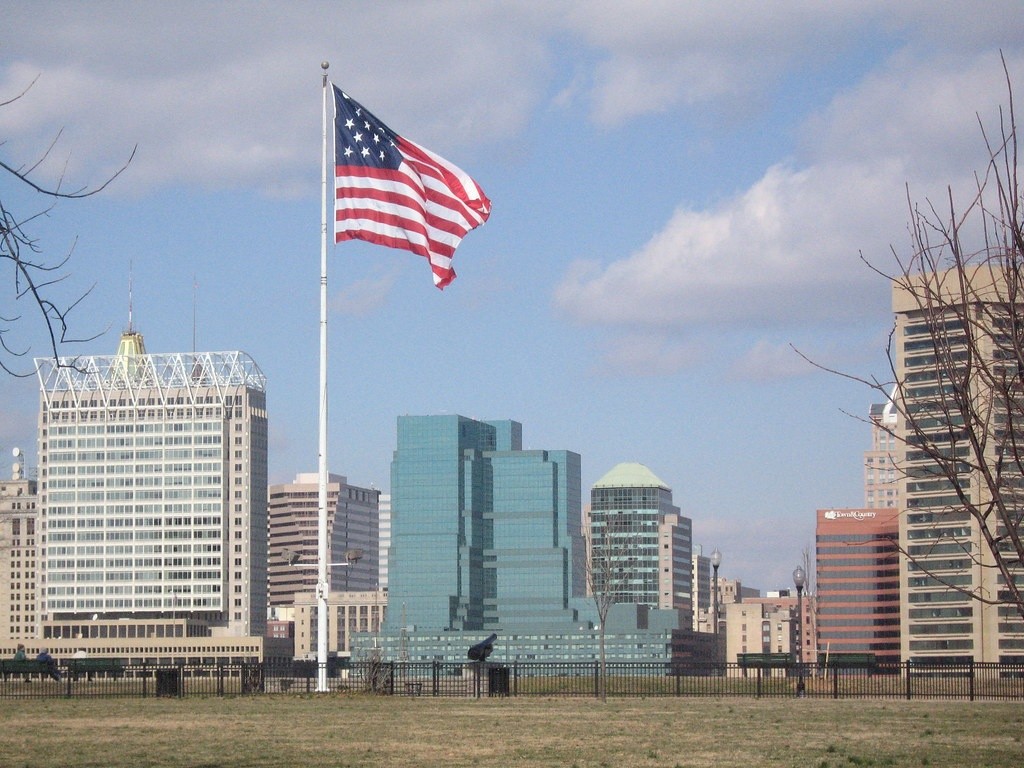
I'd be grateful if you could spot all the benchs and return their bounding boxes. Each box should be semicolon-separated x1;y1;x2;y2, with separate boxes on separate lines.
65;657;123;683
816;652;876;678
736;652;795;679
1;658;50;685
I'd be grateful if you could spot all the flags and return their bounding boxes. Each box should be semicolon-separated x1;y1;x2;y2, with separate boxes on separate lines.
332;79;493;291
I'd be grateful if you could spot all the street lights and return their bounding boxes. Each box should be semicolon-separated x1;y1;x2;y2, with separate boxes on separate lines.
793;566;806;664
711;549;722;663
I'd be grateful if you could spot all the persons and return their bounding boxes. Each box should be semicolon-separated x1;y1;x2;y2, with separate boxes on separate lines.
73;647;94;683
36;647;61;684
13;644;32;682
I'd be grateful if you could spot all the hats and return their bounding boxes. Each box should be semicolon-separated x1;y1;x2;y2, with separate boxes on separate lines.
41;646;47;653
18;644;24;650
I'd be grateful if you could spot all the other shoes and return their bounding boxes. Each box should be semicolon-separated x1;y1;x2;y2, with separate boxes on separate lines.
73;676;77;681
25;679;31;682
57;674;60;682
88;678;92;681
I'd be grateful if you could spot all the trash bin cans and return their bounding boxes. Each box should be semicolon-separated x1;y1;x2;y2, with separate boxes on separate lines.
155;667;177;698
490;668;509;697
